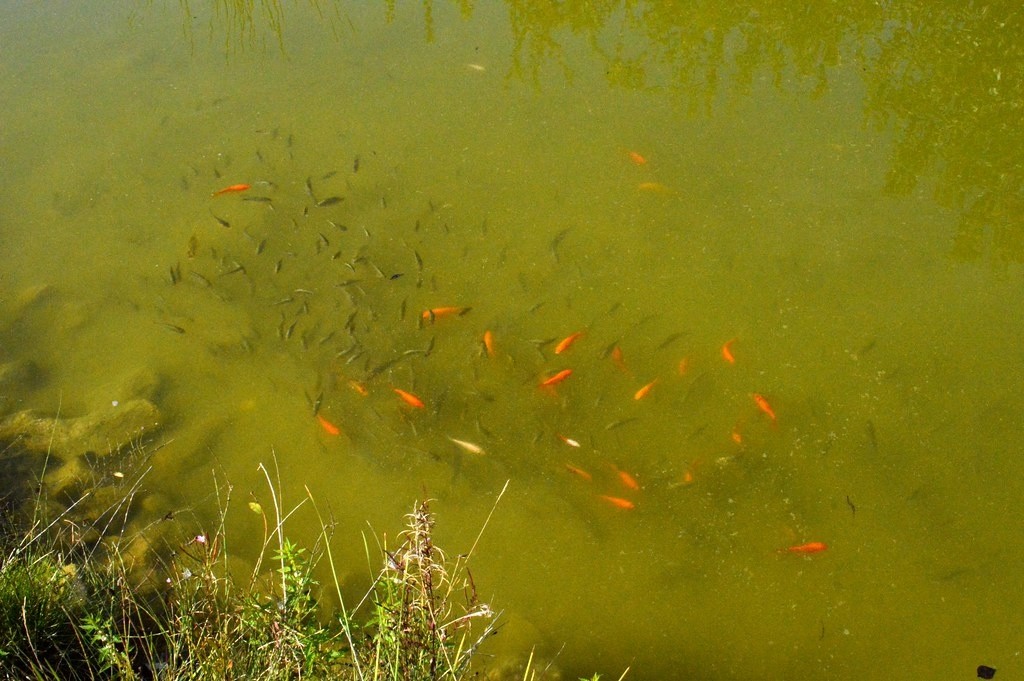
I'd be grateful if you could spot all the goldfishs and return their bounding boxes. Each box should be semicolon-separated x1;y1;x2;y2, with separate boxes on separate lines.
467;62;490;74
628;151;669;197
143;128;473;437
449;229;828;557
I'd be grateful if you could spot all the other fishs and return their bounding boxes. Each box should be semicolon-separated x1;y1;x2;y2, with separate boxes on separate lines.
844;421;970;582
